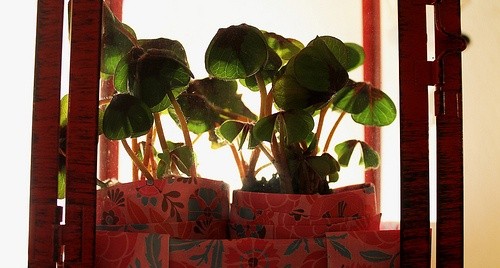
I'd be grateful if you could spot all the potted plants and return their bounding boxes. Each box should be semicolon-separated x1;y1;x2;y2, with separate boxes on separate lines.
57;0;398;243
